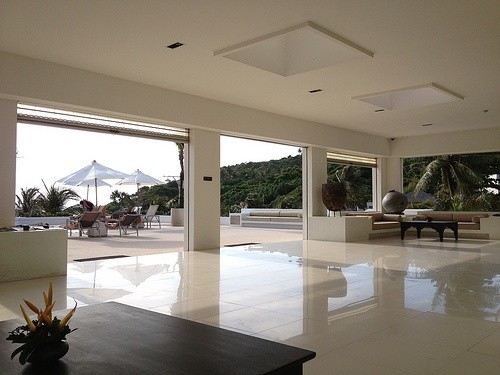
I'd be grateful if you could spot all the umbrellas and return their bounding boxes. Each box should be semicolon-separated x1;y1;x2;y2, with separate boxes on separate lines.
57;159;137;208
83;179;112;200
117;168;165;208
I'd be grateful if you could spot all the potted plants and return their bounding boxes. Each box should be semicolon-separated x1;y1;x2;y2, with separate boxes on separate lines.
4;283;78;366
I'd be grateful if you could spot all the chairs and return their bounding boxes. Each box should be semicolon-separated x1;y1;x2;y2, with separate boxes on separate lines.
66;206;161;239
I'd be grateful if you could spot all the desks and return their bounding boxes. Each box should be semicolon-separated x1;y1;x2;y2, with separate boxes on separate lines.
0;297;317;375
399;220;459;242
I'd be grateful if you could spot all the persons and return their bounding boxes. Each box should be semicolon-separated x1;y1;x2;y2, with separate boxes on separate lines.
80;200;94;212
60;207;84;229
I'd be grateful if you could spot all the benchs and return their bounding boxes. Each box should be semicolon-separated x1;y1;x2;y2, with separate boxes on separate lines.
414;210;489;230
346;211;401;229
240;208;302;230
